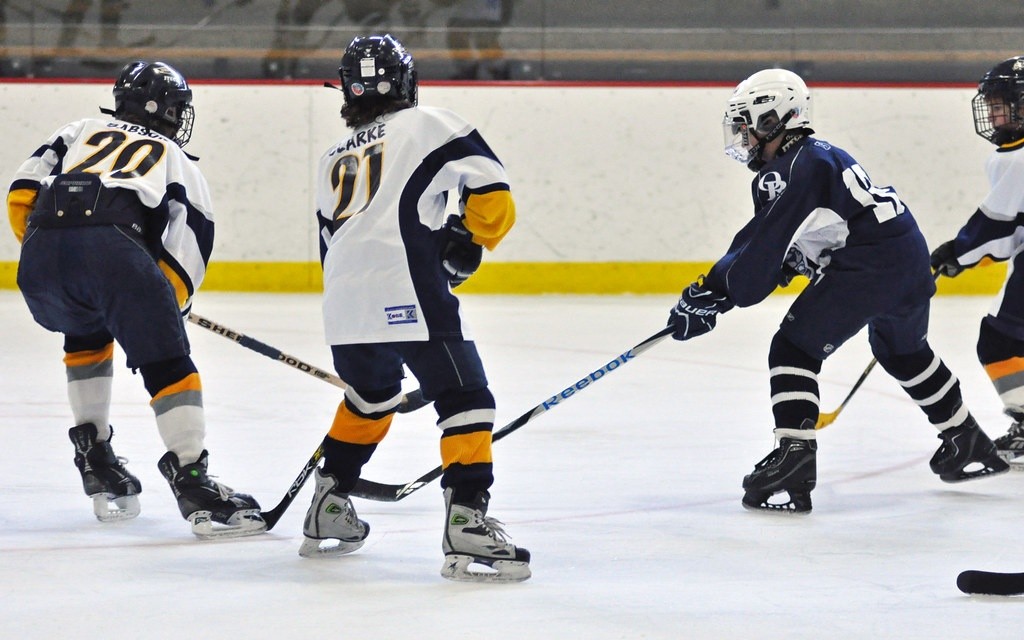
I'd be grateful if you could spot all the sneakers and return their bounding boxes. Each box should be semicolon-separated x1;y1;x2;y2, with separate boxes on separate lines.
158;449;268;540
440;486;532;584
993;405;1024;472
299;467;370;558
69;422;142;522
741;436;817;516
929;411;1010;484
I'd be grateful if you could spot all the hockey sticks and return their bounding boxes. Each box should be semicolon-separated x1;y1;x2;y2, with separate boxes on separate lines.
185;313;434;413
349;323;677;502
956;565;1024;598
260;434;329;528
817;264;944;429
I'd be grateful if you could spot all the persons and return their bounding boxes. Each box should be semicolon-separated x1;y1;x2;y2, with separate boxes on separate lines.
299;34;532;582
0;0;513;81
931;57;1024;470
667;68;1012;515
9;61;268;541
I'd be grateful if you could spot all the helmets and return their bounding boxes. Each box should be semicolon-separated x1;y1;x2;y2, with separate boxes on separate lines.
721;68;815;168
113;60;195;149
972;56;1024;146
338;34;418;107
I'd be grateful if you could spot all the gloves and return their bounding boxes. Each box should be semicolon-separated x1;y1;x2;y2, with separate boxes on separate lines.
668;282;723;341
931;239;965;278
438;215;482;288
